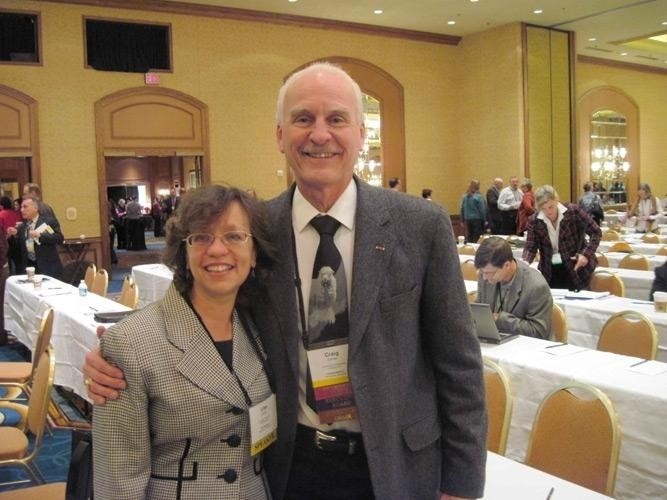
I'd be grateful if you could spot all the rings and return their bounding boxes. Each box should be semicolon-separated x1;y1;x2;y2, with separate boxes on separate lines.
82;377;91;387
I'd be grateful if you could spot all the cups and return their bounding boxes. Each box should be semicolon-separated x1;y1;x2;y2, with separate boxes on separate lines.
25;266;36;282
31;276;43;292
652;290;667;313
458;235;465;246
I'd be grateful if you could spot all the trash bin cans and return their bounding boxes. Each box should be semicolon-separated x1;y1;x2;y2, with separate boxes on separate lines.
66;428;93;499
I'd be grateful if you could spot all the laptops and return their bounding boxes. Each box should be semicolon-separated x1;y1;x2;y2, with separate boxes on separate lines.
470;303;519;344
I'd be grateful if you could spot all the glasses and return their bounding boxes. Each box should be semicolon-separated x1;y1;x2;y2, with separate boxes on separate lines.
182;230;256;247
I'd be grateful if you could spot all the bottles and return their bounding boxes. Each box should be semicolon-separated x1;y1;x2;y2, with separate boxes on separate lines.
78;279;88;310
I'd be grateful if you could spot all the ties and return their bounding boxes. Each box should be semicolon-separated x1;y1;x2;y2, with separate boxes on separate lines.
305;216;350;427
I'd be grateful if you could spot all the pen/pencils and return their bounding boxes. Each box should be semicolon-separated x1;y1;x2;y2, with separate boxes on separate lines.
631;302;655;305
631;359;649;367
48;288;62;289
89;306;99;312
546;343;568;348
57;292;72;295
547;487;555;500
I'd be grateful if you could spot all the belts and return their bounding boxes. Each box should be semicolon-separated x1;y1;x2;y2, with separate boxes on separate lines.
295;421;367;458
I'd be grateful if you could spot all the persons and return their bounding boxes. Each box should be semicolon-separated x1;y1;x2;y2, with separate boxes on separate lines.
80;62;490;500
92;182;278;499
388;175;401;189
619;183;665;234
579;183;604;228
646;259;667;303
474;235;554;339
3;182;183;279
459;178;536;242
422;187;435;200
521;183;605;291
